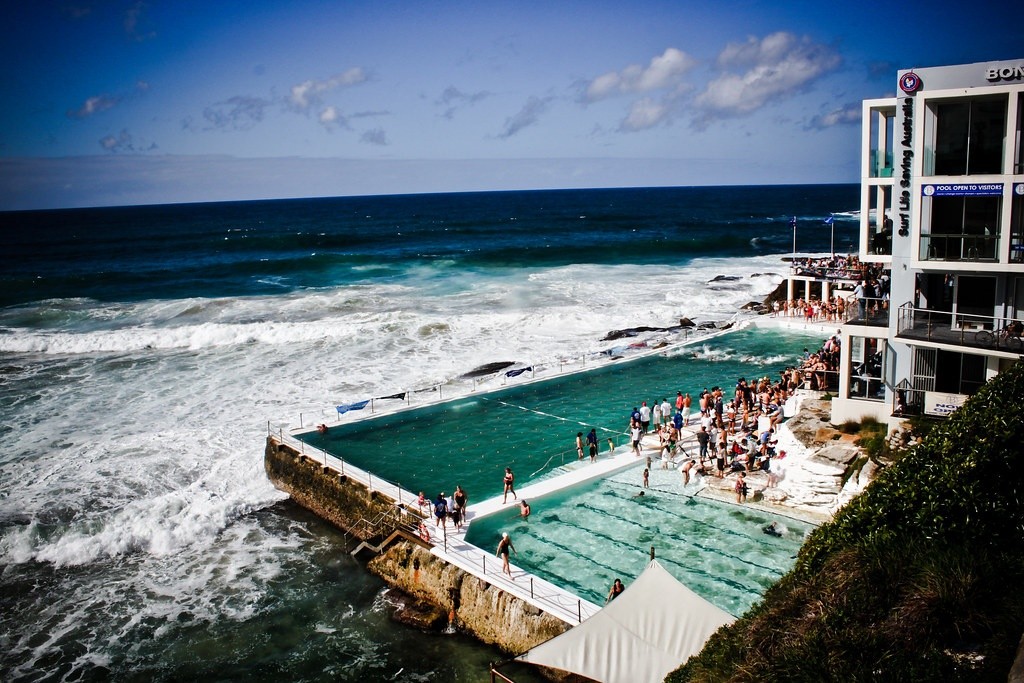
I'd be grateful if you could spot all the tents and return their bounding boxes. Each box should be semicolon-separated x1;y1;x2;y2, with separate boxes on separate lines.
486;544;745;683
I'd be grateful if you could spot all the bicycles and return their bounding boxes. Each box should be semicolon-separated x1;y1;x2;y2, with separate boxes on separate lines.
974;320;1022;352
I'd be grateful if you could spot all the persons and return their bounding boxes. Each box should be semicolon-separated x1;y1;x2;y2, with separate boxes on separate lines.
630;407;643;436
639;402;650;438
453;486;468;524
588;442;596;464
501;467;519;505
650;400;662;434
630;491;645;500
416;491;427;510
317;422;331;443
673;409;683;441
667;422;680;454
509;499;532;525
762;520;784;539
659;398;672;428
576;432;584;462
1000;317;1023;342
646;456;654;471
603;577;626;605
878;214;893;242
681;459;696;490
630;424;641;455
738;481;752;502
674;389;683;419
660;429;670;449
432;494;449;531
633;422;643;454
450;501;462;534
643;469;650;488
681;393;692;427
661;441;673;470
607;437;615;457
494;532;520;581
585;427;600;457
733;471;747;504
693;251;917;486
